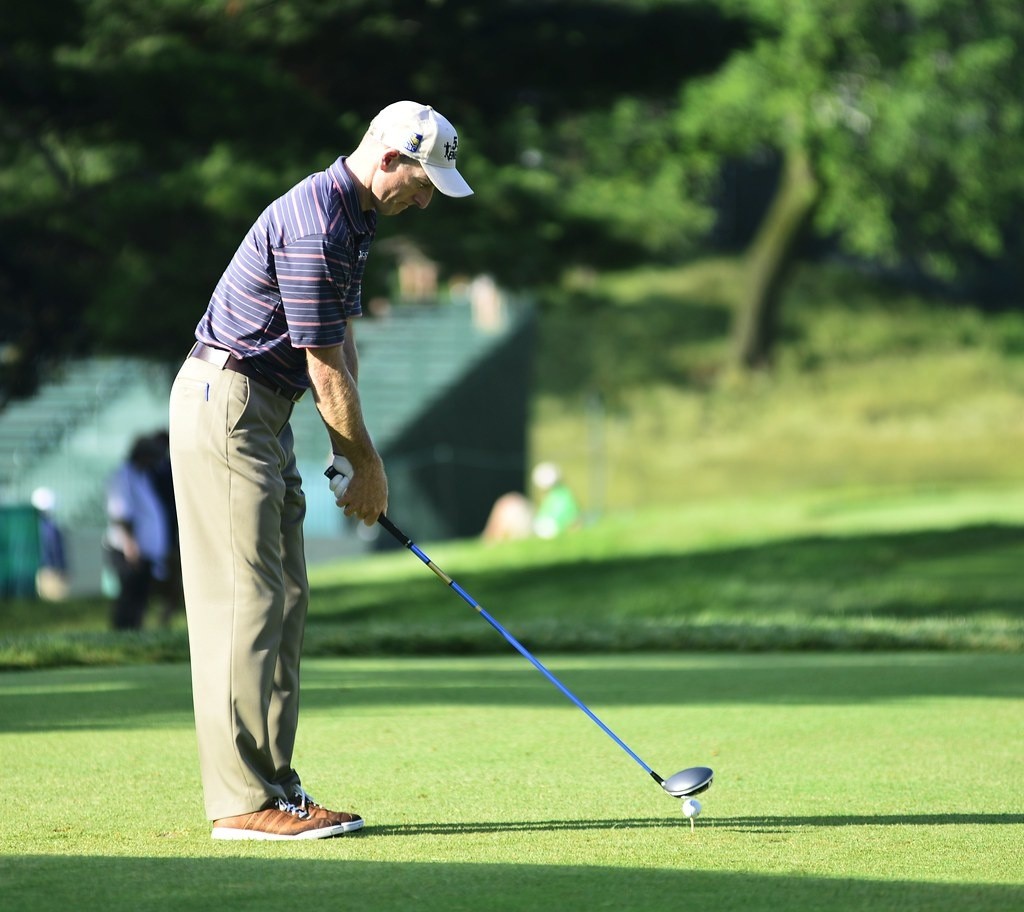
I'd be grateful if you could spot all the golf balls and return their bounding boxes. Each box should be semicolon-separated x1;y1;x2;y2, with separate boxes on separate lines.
681;798;702;818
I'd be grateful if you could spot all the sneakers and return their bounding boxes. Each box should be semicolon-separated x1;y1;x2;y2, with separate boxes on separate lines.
211;783;364;840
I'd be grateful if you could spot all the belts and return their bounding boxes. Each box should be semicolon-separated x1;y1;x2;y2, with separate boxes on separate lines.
191;341;308;401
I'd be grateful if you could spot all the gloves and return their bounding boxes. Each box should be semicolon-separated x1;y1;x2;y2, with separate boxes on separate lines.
329;455;355;498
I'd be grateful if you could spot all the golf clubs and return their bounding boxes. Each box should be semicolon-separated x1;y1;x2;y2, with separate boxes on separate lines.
323;462;715;800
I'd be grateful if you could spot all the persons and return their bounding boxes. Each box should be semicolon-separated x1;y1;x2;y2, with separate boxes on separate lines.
166;99;476;844
104;439;167;631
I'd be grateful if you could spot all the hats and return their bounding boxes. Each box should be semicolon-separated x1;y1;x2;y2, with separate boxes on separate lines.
369;101;474;198
